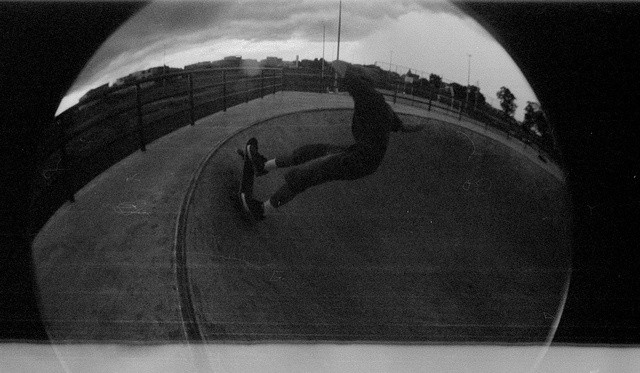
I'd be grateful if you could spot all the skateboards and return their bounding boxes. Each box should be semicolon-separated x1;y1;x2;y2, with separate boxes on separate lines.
230;138;258;223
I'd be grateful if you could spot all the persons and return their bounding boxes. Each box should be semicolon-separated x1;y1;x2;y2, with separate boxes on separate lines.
239;64;427;222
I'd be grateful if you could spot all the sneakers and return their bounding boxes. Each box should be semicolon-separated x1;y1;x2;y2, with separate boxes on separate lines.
240;190;266;223
246;143;269;177
